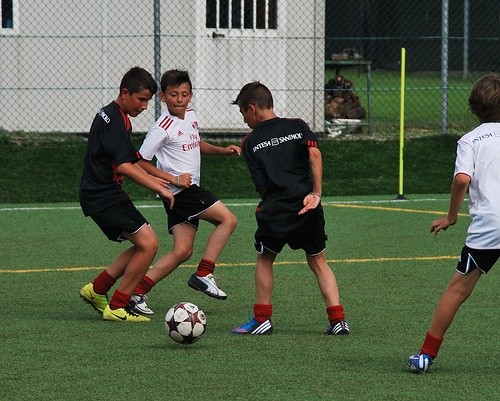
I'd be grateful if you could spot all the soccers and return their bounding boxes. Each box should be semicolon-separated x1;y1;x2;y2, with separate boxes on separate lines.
165;301;207;345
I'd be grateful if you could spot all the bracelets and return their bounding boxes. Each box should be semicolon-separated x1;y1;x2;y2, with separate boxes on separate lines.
309;192;322;198
177;176;180;186
171;176;176;185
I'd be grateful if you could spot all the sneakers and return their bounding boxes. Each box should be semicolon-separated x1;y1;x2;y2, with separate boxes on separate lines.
187;273;228;300
131;294;154;315
327;320;349;335
102;305;150;322
79;281;109;313
408;351;433;374
232;311;272;335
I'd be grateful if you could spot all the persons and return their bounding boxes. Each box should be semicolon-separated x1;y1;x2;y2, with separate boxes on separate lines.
403;73;500;375
231;82;350;336
126;69;241;315
78;65;174;322
